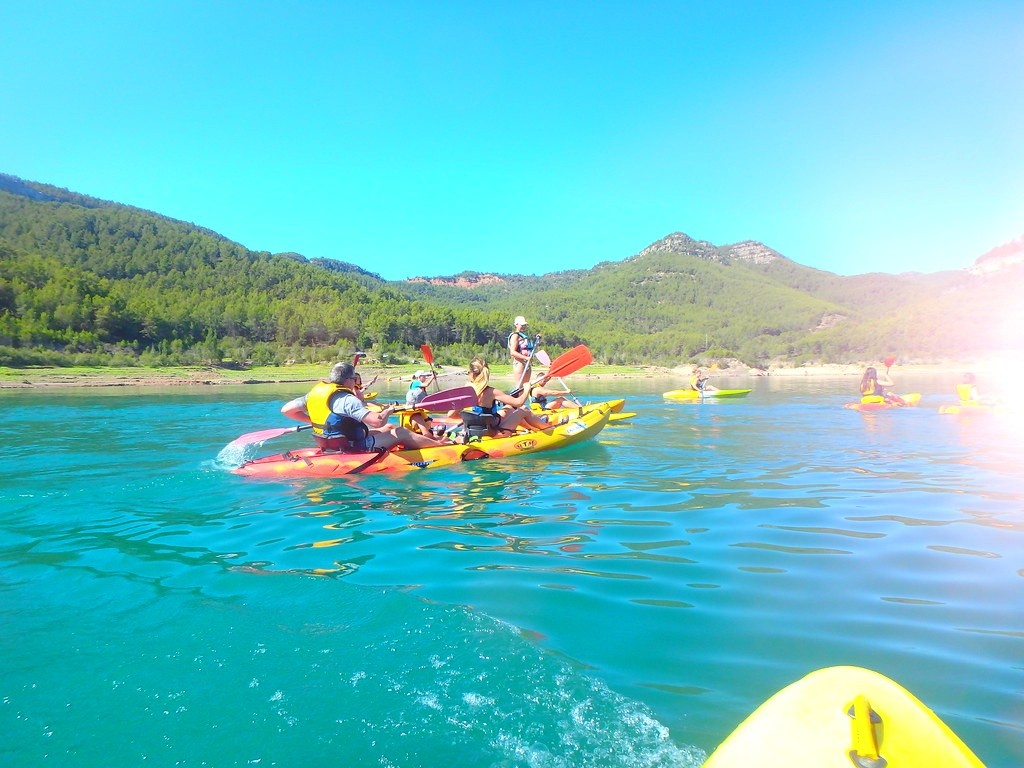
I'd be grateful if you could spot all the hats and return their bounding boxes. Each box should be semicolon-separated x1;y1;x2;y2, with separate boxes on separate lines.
514;316;528;326
414;370;432;378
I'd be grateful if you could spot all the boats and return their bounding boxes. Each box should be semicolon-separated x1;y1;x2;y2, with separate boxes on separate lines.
230;392;639;478
936;399;998;415
845;392;922;410
661;386;752;398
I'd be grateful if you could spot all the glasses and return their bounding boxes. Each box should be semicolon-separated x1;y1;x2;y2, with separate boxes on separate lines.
346;377;357;382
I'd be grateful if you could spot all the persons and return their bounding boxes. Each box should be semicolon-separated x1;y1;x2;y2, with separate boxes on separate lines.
690;370;719;392
279;315;571;451
860;368;895;396
957;372;980;402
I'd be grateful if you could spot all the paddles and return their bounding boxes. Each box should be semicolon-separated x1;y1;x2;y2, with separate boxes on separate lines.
535;349;582;409
237;385;478;446
353;354;359;369
695;364;717;399
445;343;593;434
883;355;896;394
364;375;379;390
511;336;541;397
420;344;440;392
